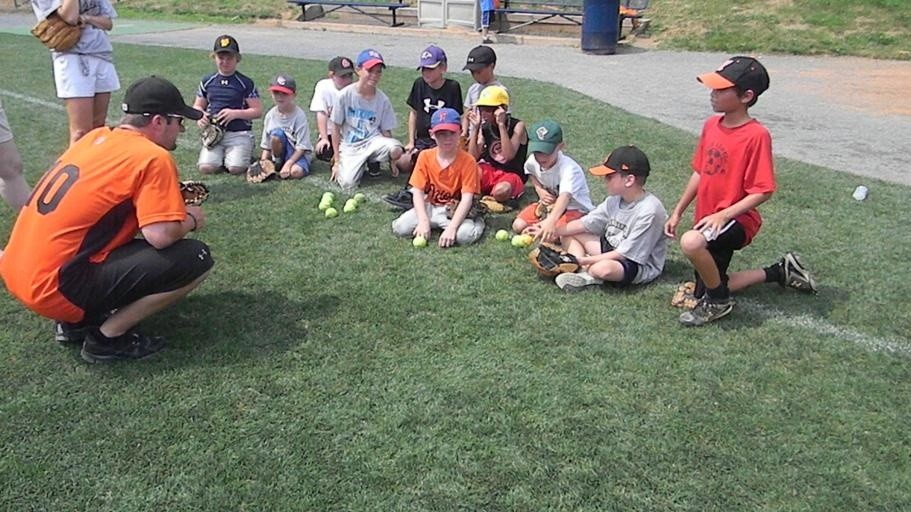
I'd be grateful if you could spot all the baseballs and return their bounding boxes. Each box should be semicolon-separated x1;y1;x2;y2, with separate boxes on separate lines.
413;237;428;249
344;193;364;213
318;191;338;218
495;230;533;248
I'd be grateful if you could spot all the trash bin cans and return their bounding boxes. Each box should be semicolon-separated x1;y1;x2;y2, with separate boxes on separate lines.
581;0;620;55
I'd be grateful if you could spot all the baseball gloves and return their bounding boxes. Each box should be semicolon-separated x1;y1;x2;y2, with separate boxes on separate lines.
177;180;209;207
671;281;707;309
529;243;580;277
200;116;225;151
445;198;487;219
247;159;276;183
30;14;87;52
480;195;517;214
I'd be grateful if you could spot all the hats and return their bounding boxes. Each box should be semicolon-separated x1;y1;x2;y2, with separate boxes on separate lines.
526;119;563;155
358;48;385;69
329;56;355;78
470;85;510;108
587;146;650;176
697;55;770;94
267;74;297;95
416;46;447;69
463;45;496;72
213;34;239;55
429;108;463;134
121;74;204;120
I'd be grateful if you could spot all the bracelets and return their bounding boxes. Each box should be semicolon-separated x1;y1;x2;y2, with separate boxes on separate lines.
186;212;197;232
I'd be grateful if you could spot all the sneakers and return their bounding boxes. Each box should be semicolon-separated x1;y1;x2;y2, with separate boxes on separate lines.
778;250;816;294
554;270;603;291
56;311;166;364
382;187;414;209
679;293;733;327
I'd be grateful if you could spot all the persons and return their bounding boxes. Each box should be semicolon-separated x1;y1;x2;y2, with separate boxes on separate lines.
477;0;501;43
329;48;407;192
0;75;215;366
468;83;532;203
0;103;34;212
260;71;315;181
461;44;512;152
390;106;486;248
511;115;596;237
189;34;266;175
30;1;121;150
521;143;672;292
309;56;357;163
394;44;465;174
662;56;818;327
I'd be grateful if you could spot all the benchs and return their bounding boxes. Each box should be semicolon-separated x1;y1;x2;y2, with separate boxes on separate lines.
494;9;644;41
287;0;410;28
